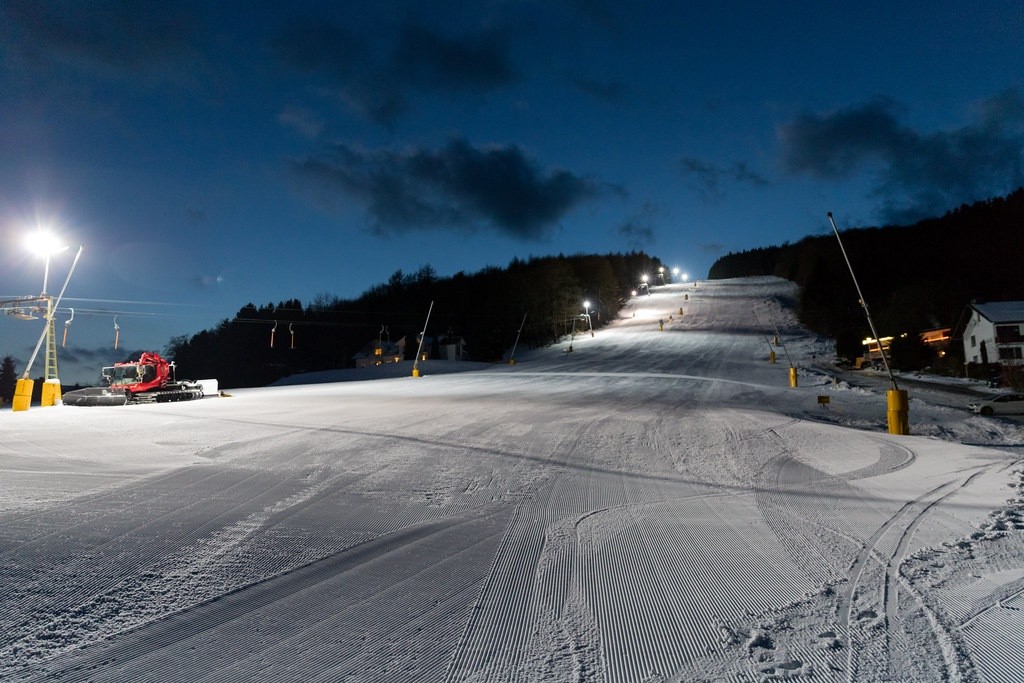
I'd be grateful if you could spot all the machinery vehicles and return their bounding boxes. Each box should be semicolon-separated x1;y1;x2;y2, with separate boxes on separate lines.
62;351;204;406
